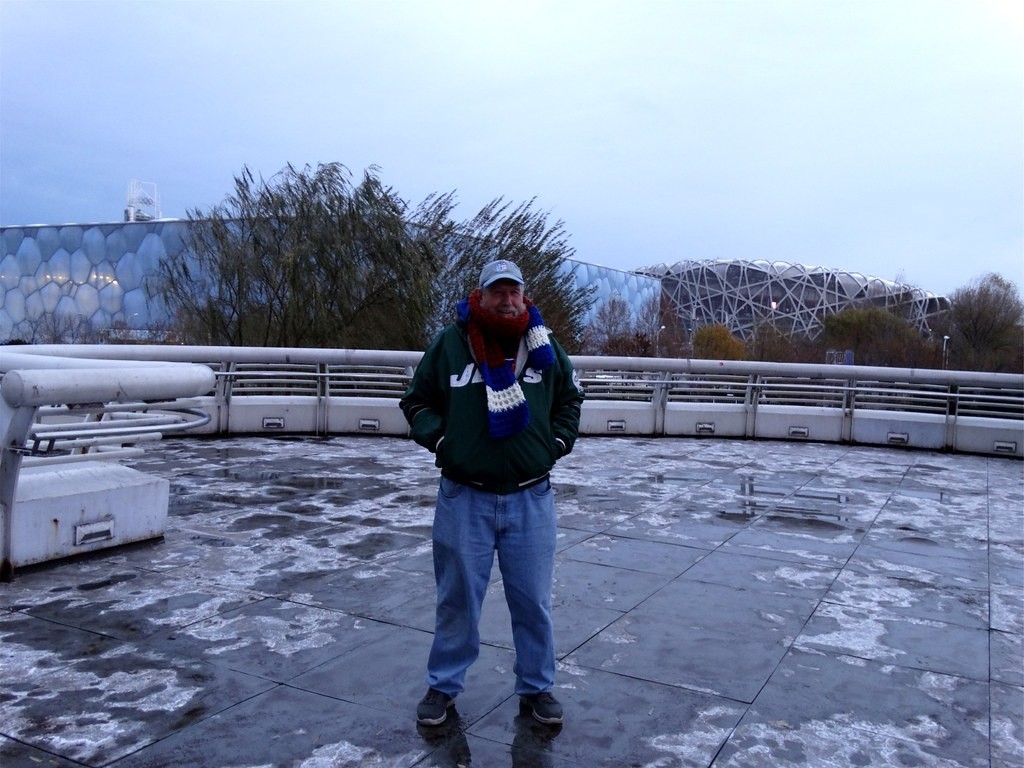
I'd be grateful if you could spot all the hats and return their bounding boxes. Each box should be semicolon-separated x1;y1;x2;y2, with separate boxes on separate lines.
479;260;524;288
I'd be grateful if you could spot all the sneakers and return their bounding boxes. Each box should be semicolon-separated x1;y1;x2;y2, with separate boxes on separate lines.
521;689;564;724
415;687;457;726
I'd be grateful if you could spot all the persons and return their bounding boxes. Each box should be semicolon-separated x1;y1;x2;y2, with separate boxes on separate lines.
398;261;586;725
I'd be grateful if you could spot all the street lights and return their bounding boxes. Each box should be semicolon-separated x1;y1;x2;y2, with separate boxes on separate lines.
943;335;950;360
657;325;667;346
